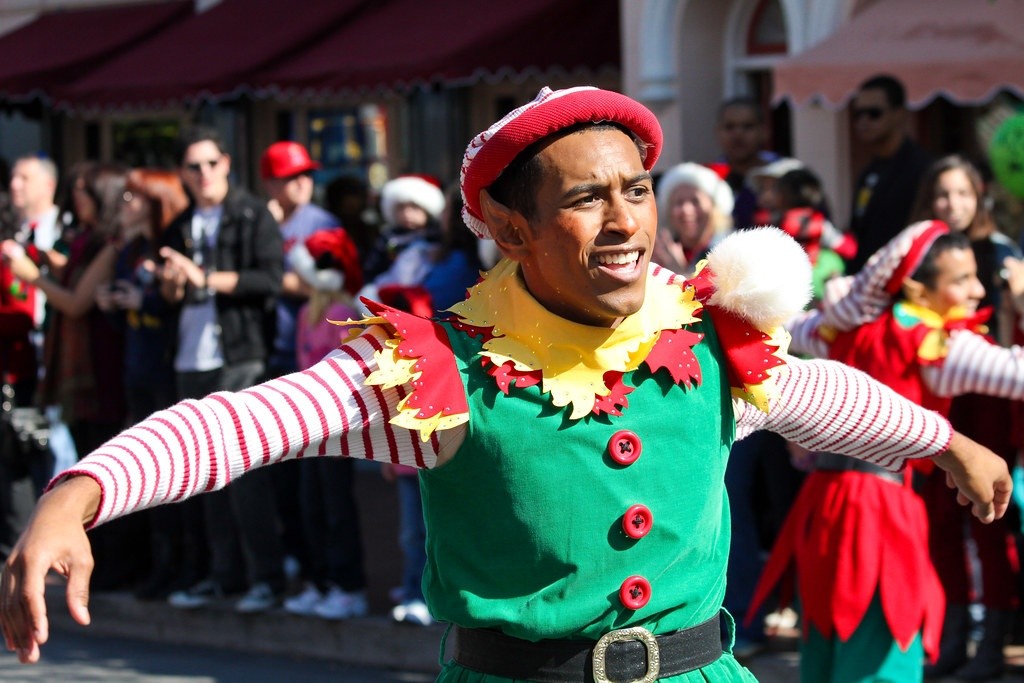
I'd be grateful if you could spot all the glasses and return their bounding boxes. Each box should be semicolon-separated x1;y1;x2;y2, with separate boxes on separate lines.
848;103;898;119
178;155;225;172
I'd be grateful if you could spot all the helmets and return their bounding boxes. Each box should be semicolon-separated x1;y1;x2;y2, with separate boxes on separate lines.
254;141;320;181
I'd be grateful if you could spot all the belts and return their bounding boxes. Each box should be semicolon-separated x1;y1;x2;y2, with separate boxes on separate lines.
442;612;723;683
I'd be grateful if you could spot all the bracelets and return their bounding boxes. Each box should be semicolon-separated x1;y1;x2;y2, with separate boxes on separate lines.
202;265;216;288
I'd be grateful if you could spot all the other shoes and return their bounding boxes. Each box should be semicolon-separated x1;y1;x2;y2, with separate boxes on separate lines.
95;571;435;628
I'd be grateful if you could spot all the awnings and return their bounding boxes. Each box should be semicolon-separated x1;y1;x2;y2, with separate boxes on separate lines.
0;0;621;118
769;0;1024;113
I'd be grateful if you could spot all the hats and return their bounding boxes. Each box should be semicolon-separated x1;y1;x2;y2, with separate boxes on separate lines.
460;84;664;242
380;171;447;227
744;157;835;220
287;227;363;296
656;160;736;233
820;217;952;335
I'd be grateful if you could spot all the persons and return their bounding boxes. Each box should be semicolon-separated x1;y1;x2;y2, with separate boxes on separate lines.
654;75;1024;683
0;86;1013;683
0;128;501;625
